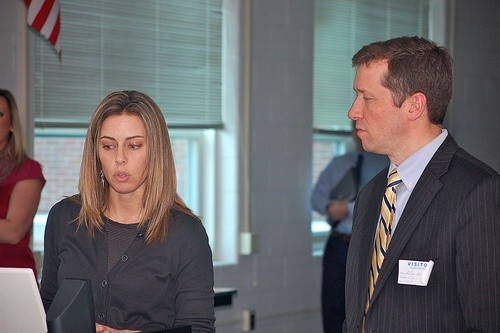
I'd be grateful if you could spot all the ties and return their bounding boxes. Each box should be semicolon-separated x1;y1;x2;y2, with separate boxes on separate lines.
361;168;403;333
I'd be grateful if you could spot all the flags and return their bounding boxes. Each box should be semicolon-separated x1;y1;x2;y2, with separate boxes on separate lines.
24;0;65;64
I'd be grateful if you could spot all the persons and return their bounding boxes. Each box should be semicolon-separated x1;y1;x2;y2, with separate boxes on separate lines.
341;37;500;333
42;90;216;333
0;89;46;292
310;121;389;333
